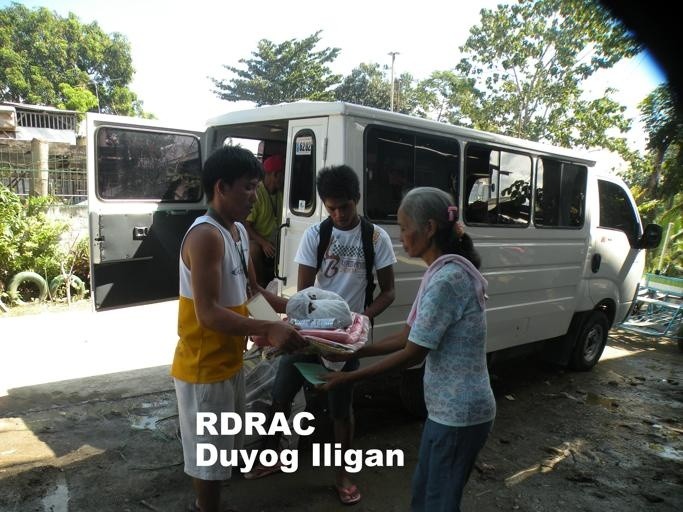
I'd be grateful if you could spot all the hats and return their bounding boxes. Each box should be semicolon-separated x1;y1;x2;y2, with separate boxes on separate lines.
263;155;285;173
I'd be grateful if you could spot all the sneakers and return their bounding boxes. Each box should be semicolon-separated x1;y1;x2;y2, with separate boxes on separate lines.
188;496;203;512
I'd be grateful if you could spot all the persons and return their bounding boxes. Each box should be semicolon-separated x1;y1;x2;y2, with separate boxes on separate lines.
241;152;285;274
322;188;496;512
243;169;399;504
166;144;310;511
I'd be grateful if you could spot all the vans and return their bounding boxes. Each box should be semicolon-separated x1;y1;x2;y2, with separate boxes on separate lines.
83;99;663;421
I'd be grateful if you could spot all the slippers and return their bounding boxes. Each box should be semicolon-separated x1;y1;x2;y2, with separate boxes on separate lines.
243;458;287;480
335;483;361;505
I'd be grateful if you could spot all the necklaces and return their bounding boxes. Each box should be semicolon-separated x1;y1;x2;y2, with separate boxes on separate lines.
267;187;279;224
